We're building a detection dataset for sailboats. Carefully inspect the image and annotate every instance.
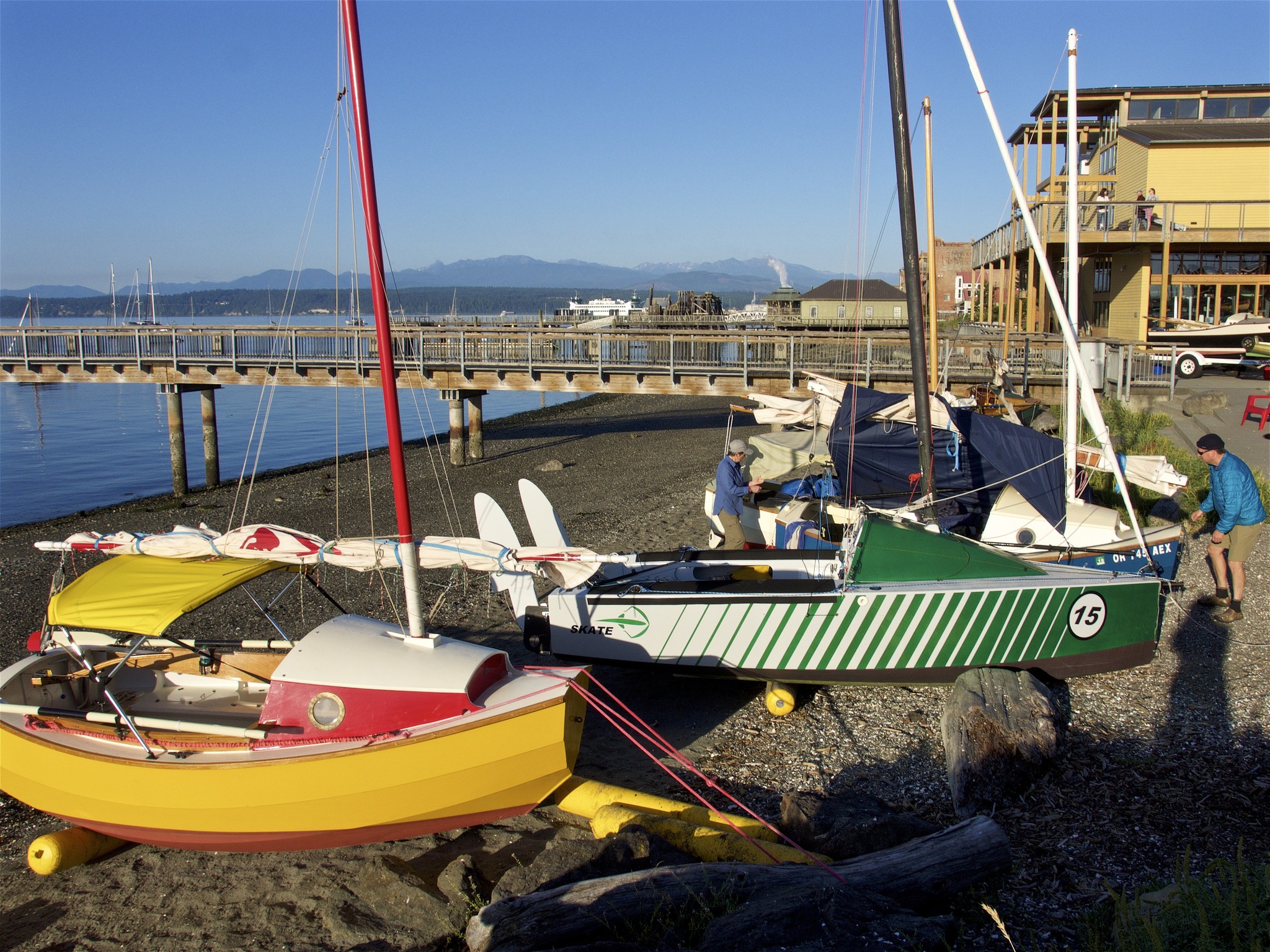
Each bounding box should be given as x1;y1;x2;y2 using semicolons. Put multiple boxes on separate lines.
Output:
0;0;595;854
469;0;1165;687
700;2;1187;594
6;257;183;354
343;269;367;326
188;296;202;334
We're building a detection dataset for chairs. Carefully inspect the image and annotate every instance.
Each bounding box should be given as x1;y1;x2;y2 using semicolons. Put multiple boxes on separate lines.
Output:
1240;395;1270;430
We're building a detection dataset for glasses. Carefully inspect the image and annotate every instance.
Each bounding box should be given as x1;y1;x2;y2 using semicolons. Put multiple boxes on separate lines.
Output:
1197;449;1211;455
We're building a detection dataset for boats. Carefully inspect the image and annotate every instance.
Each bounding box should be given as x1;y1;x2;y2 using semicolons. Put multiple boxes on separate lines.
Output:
382;275;645;329
1147;312;1270;348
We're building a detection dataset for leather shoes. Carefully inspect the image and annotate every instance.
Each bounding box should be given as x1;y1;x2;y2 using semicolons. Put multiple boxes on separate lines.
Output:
1197;594;1232;607
1213;608;1243;623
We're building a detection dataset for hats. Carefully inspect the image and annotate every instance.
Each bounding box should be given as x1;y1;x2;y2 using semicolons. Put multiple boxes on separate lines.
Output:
1196;433;1225;450
728;440;754;455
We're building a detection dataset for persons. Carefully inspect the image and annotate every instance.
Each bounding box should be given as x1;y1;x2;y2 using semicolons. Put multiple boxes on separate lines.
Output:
1133;189;1149;231
711;439;764;550
1189;432;1267;623
1095;188;1110;230
1142;187;1159;231
